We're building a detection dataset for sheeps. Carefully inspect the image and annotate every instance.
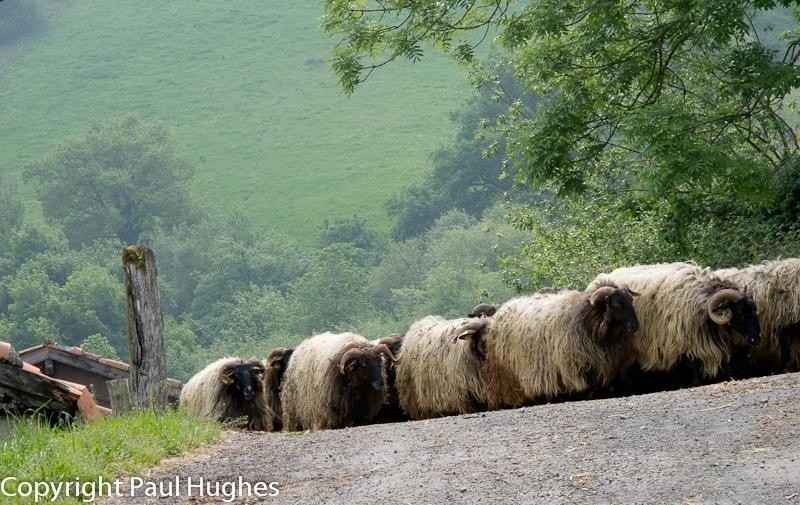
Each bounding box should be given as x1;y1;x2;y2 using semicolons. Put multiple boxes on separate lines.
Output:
278;330;405;438
483;260;641;414
609;258;800;397
176;346;293;436
394;302;497;421
